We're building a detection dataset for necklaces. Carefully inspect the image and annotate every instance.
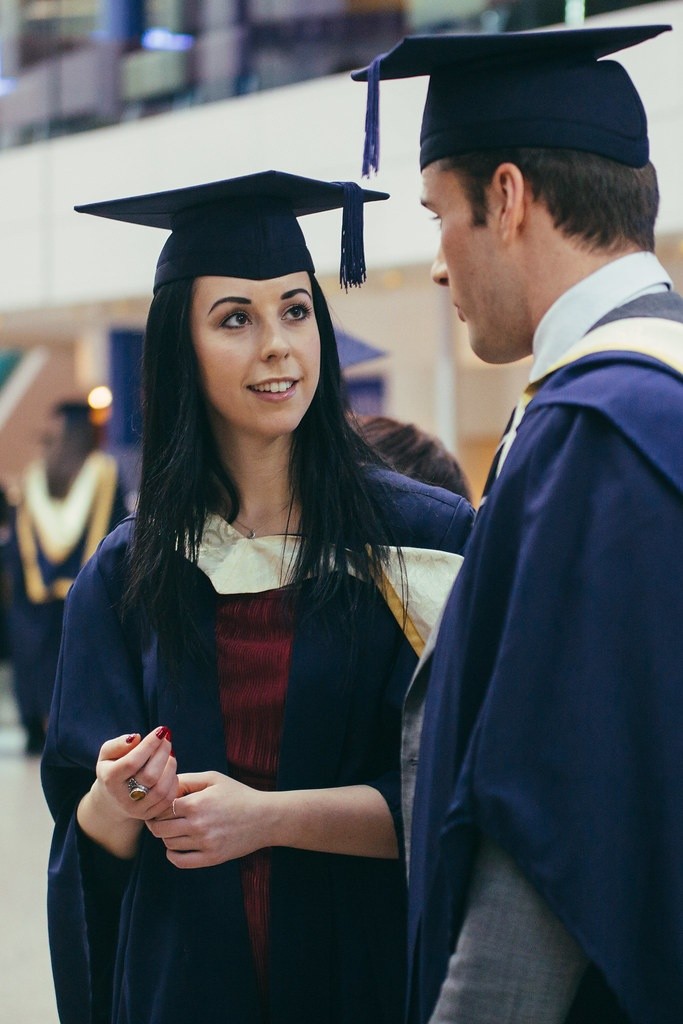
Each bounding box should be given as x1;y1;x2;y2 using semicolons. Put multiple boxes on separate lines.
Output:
236;500;290;538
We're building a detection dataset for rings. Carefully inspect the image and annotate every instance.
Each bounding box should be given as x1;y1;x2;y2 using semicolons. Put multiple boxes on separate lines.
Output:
172;797;177;816
127;778;150;802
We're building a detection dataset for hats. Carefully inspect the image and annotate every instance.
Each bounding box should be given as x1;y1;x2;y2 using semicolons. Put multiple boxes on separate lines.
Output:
75;169;390;294
351;24;672;179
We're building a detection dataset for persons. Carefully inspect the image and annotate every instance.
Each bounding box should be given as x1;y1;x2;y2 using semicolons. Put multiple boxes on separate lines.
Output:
38;172;476;1024
0;394;128;752
349;416;469;507
350;26;683;1024
